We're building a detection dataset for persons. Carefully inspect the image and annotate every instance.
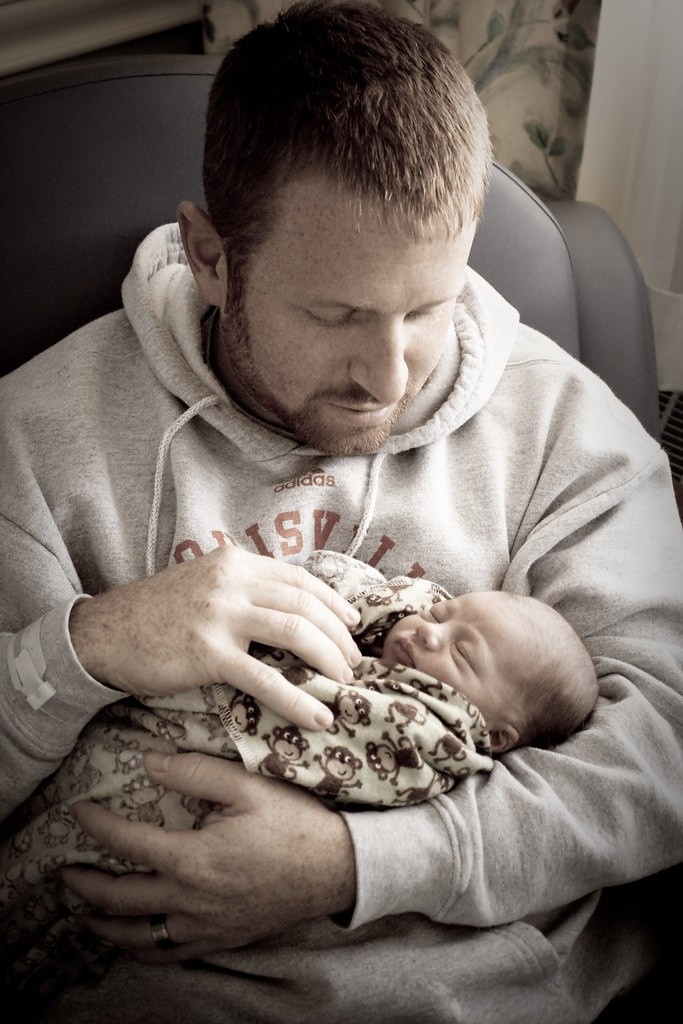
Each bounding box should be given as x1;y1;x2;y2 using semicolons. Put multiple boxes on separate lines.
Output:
1;0;683;1024
1;552;599;996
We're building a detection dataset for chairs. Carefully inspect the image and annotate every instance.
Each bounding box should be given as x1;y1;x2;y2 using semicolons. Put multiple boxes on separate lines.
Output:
0;53;658;446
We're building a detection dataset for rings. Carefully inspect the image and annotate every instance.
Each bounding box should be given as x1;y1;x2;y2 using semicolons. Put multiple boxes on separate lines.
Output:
150;915;183;949
